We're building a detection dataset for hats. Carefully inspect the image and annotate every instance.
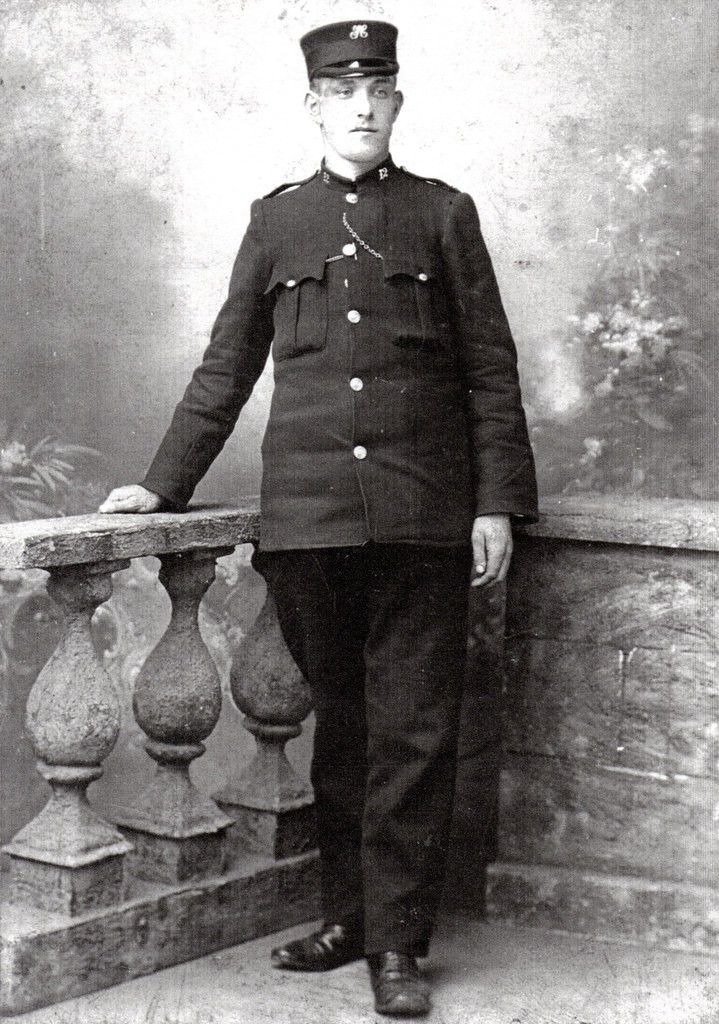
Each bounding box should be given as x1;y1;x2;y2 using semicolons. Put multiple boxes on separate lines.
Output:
299;20;400;82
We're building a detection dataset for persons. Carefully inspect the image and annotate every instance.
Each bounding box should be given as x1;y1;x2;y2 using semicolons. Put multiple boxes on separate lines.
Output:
99;21;542;1017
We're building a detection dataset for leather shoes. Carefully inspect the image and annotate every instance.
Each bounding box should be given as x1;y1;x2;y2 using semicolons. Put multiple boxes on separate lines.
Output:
365;952;430;1017
270;923;366;973
